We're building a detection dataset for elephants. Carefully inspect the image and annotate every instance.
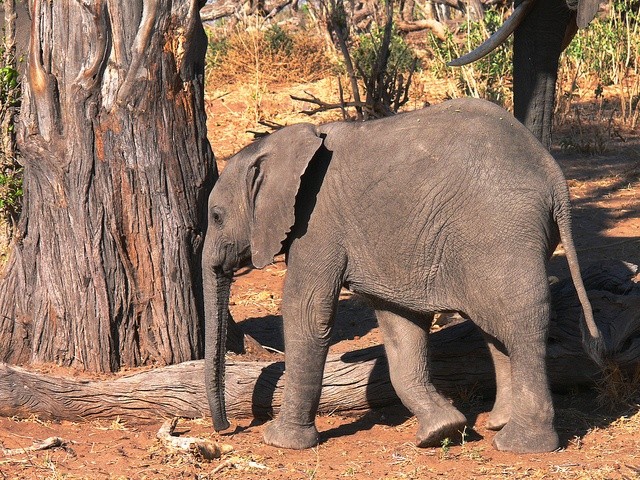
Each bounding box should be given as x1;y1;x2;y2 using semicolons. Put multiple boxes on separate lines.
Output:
200;96;609;454
